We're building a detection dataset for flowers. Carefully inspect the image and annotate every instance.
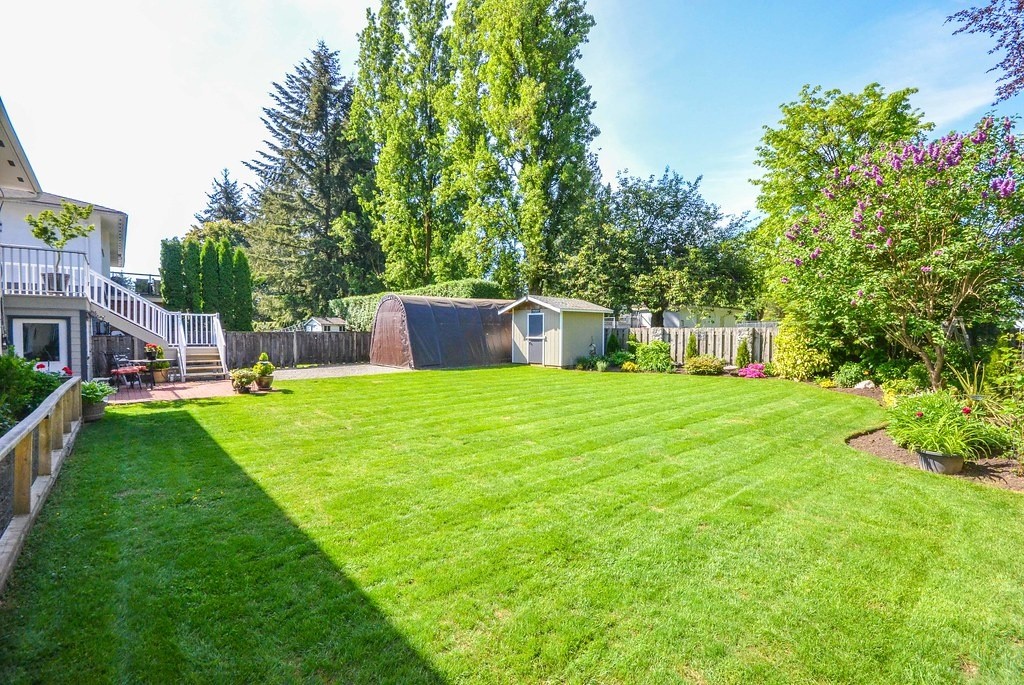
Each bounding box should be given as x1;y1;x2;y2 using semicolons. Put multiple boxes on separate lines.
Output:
144;343;161;354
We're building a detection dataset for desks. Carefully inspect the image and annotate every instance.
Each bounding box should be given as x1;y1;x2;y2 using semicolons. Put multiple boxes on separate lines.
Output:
128;359;178;390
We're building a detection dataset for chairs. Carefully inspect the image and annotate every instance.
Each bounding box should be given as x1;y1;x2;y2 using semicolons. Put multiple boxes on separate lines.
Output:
110;352;141;391
116;354;147;389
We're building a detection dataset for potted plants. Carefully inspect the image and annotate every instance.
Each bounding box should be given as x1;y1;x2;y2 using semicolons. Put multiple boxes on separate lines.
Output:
23;198;96;291
79;380;118;421
227;368;241;391
232;368;255;394
896;412;1002;476
146;361;171;383
253;353;275;391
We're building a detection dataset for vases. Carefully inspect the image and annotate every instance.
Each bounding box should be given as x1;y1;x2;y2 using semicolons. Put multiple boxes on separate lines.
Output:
147;351;158;360
